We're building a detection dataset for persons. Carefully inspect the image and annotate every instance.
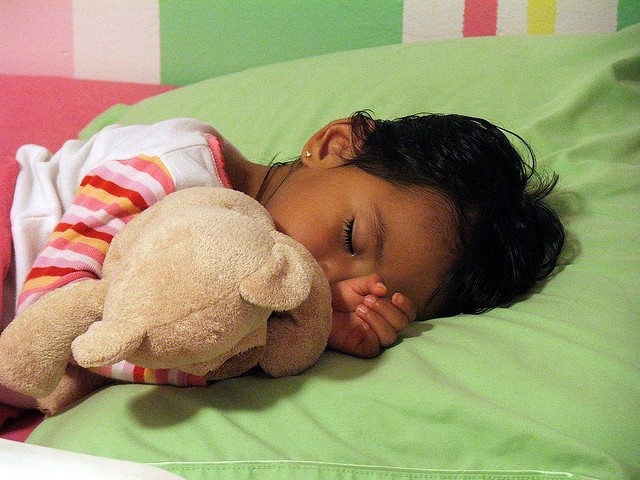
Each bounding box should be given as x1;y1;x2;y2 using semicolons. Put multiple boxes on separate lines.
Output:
1;109;566;421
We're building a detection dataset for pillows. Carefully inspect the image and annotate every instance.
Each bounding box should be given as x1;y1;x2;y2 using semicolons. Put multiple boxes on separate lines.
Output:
23;24;639;480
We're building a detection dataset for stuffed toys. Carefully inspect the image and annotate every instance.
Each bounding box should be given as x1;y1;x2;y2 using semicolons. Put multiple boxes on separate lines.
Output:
0;186;333;416
